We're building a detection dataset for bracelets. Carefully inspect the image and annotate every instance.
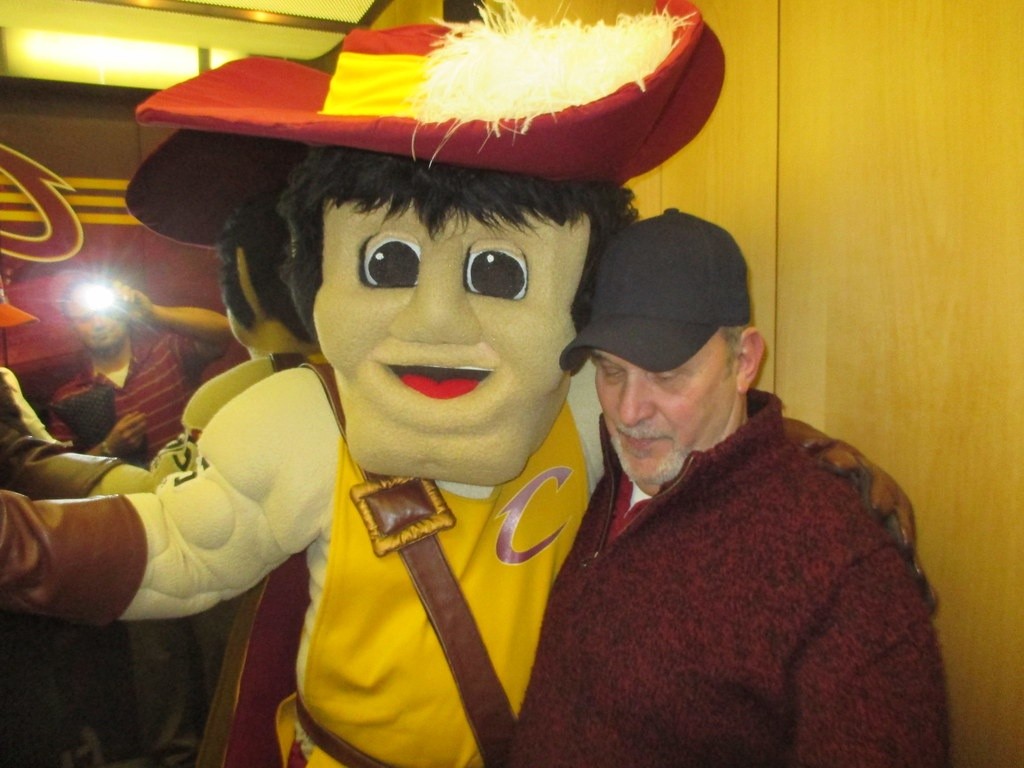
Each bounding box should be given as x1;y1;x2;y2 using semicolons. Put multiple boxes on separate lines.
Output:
100;440;114;457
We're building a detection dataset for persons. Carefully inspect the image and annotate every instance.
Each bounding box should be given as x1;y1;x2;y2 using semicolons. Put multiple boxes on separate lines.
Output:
0;119;321;765
45;260;230;764
505;206;958;768
0;1;925;768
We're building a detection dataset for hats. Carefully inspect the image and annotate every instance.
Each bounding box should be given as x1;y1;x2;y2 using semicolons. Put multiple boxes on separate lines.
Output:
560;208;751;377
